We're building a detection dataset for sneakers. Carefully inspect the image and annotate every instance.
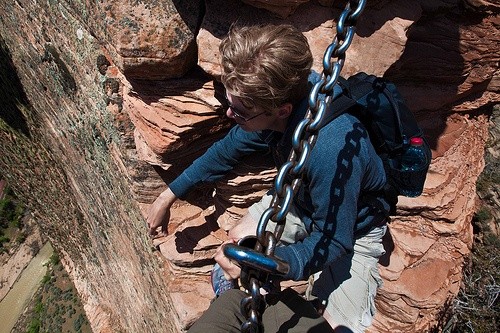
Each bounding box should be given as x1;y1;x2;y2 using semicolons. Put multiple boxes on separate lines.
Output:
210;262;238;297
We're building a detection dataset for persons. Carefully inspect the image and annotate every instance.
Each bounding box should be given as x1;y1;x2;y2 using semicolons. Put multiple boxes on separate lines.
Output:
186;263;335;333
145;21;391;333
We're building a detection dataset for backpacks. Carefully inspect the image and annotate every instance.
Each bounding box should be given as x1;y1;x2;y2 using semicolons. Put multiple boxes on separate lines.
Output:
318;73;432;197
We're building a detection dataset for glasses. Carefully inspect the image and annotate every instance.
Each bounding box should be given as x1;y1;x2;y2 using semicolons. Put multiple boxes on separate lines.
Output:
225;96;266;122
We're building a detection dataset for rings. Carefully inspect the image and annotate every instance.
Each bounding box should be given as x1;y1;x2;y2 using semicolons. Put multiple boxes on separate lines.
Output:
148;223;150;228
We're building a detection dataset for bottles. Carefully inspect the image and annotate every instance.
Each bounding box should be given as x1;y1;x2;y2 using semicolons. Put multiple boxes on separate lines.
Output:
400;138;427;198
